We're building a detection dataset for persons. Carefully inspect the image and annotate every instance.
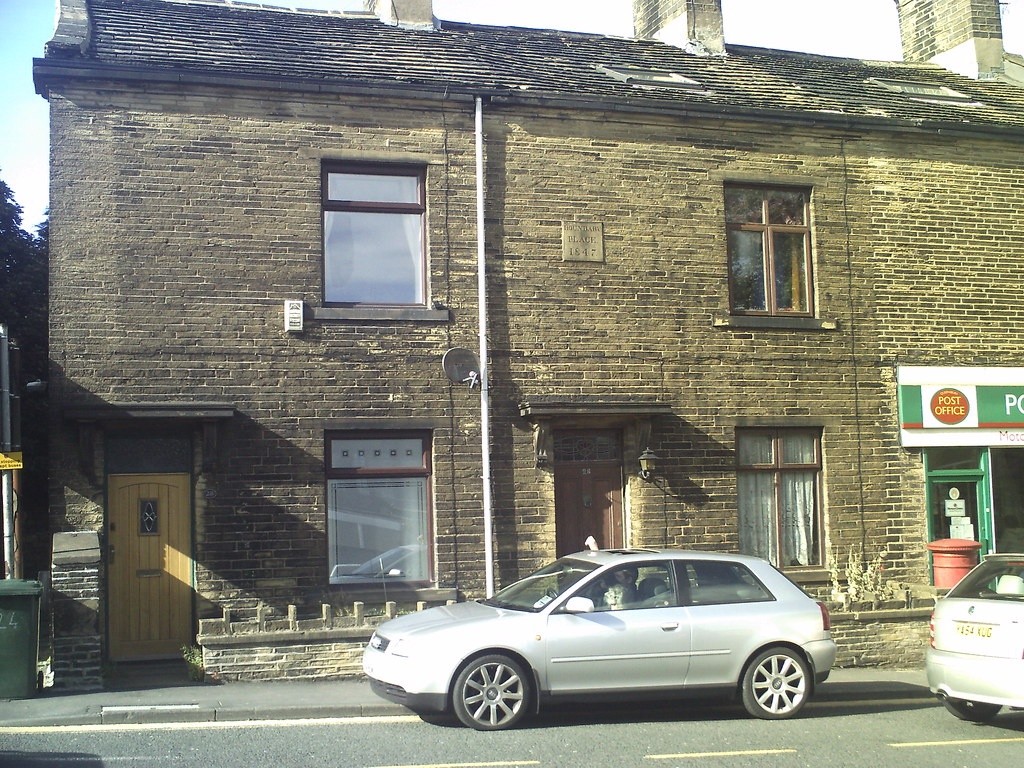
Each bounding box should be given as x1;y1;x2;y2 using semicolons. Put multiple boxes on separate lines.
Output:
996;515;1024;553
615;566;638;603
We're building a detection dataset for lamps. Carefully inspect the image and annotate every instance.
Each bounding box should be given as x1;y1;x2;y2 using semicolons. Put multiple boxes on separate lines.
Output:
638;446;663;480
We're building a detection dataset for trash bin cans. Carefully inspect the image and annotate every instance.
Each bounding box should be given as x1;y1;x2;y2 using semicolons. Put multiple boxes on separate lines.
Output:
0;579;44;698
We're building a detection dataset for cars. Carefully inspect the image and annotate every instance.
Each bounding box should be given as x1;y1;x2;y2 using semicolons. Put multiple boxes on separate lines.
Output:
362;547;836;731
924;552;1024;719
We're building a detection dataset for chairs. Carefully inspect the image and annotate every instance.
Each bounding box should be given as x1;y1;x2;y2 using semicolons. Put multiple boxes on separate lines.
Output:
634;578;668;607
603;569;639;605
